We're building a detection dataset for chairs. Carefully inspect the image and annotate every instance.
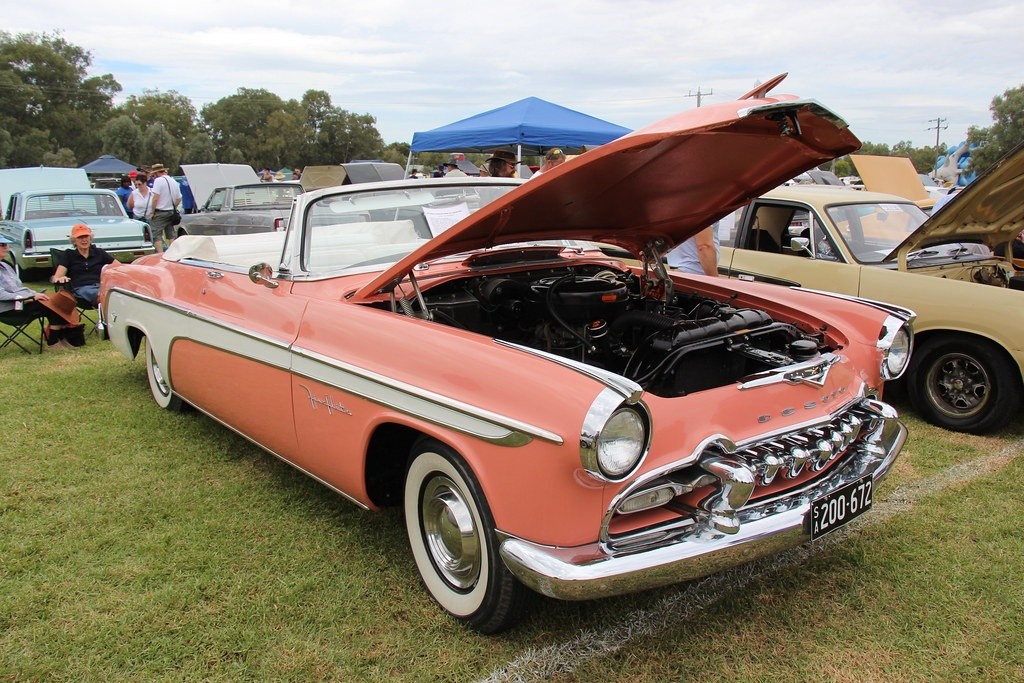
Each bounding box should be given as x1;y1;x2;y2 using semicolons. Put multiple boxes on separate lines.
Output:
748;229;781;253
0;288;47;355
50;244;96;336
274;197;296;206
801;227;829;256
234;199;252;208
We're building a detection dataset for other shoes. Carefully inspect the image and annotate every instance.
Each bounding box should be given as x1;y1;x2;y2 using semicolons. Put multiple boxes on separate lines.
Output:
50;338;75;349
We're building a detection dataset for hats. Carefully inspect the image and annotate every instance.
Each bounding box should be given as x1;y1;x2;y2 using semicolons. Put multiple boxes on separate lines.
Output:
484;151;524;165
0;234;15;243
443;157;458;168
72;224;91;239
150;164;170;174
36;291;79;324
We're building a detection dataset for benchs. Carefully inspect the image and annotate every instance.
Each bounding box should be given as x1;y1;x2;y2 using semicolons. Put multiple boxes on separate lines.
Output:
163;220;432;274
26;209;96;220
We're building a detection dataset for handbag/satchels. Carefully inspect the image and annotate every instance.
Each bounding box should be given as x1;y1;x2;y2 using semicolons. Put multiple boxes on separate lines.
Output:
138;217;146;222
171;212;181;225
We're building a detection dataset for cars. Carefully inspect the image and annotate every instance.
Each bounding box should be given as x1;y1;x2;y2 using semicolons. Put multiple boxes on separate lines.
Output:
0;161;480;283
716;141;1024;435
97;73;917;636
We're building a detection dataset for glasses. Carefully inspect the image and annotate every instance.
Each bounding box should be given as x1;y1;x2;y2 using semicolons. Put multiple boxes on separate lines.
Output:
548;149;563;159
0;243;6;247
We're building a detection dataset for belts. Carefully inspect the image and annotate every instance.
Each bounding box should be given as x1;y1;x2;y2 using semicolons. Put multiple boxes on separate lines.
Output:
155;208;174;212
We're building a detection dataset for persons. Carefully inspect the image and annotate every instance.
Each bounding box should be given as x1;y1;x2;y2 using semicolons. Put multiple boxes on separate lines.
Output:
409;169;418;179
0;235;74;351
443;159;468;177
129;165;154;189
293;173;298;180
51;224;123;307
438;164;445;177
434;172;440;177
127;174;153;223
116;176;134;219
149;164;182;253
668;219;720;277
485;150;524;178
530;148;566;179
261;168;272;182
293;168;301;179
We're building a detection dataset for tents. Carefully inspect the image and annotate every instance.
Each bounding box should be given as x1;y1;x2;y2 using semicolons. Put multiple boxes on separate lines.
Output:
257;169;276;175
280;167;294;174
391;96;636;221
80;155;139;173
